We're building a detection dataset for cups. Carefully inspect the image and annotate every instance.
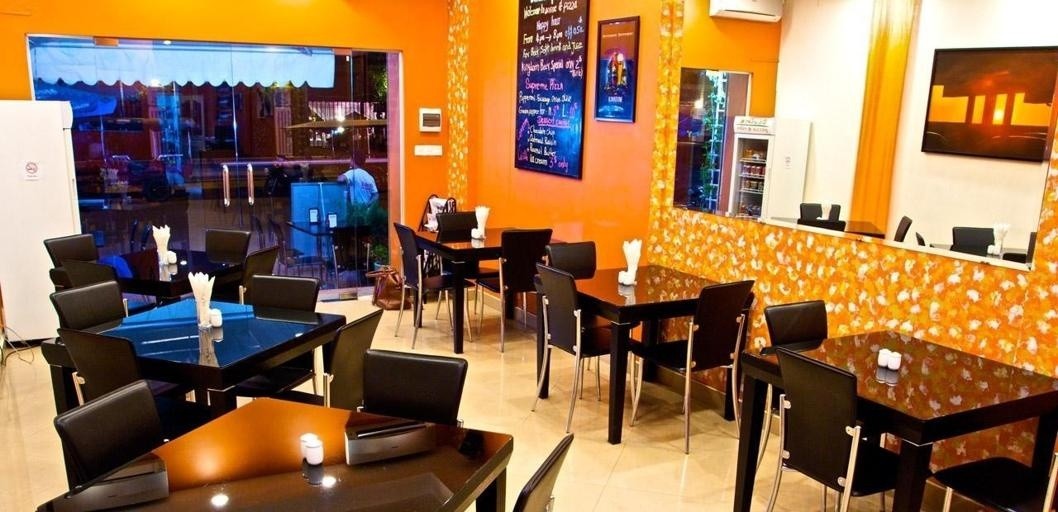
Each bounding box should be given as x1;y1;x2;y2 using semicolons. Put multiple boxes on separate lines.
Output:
470;222;488;239
298;432;330;469
195;298;221;329
158;250;176;265
618;259;640;287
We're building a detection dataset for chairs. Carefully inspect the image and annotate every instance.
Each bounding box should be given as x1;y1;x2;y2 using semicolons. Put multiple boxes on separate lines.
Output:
532;241;756;455
734;298;1057;511
31;199;574;512
798;202;1037;266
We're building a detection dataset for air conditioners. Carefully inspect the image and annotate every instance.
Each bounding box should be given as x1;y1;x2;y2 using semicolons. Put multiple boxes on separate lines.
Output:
708;0;784;25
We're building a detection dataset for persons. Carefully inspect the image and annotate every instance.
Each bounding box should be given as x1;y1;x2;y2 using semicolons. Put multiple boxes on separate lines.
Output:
338;144;380;208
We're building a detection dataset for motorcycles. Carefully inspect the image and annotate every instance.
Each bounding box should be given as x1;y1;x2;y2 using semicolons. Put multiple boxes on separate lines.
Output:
262;151;314;196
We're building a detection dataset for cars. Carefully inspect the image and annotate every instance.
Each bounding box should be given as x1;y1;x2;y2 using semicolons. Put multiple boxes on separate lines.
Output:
158;153;191;166
109;153;133;165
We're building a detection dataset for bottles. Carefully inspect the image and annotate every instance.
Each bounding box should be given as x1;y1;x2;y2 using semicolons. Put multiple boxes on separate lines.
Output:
877;349;901;370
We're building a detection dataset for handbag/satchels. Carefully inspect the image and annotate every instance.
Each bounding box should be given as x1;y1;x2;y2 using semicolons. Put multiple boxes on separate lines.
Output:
371;263;412;311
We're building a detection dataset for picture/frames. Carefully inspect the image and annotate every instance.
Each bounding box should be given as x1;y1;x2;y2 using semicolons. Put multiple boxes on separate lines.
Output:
919;43;1057;164
593;13;641;124
